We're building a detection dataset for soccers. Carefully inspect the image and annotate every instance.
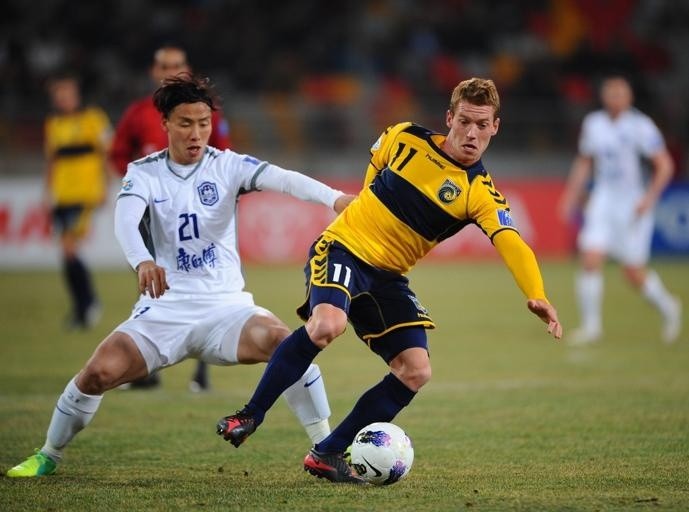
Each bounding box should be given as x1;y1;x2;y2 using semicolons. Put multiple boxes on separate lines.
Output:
351;422;414;484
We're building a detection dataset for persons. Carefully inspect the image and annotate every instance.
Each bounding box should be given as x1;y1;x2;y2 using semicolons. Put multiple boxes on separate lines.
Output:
557;78;682;342
216;77;562;483
6;72;360;478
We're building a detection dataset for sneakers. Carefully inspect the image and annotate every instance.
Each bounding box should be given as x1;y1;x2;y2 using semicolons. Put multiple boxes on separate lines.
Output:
304;448;365;482
216;410;257;448
6;448;59;477
